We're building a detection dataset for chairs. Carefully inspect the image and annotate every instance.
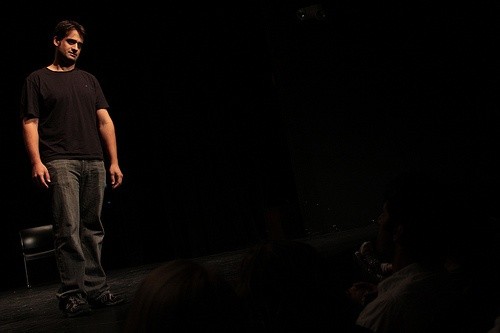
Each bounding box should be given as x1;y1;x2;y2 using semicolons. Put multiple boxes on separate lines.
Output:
20;224;62;288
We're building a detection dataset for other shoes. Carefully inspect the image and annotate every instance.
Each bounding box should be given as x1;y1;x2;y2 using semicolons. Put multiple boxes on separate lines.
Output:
58;293;94;318
89;289;124;309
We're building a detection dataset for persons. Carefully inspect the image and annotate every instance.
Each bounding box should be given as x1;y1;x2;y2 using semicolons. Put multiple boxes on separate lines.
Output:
125;187;462;333
24;20;123;316
